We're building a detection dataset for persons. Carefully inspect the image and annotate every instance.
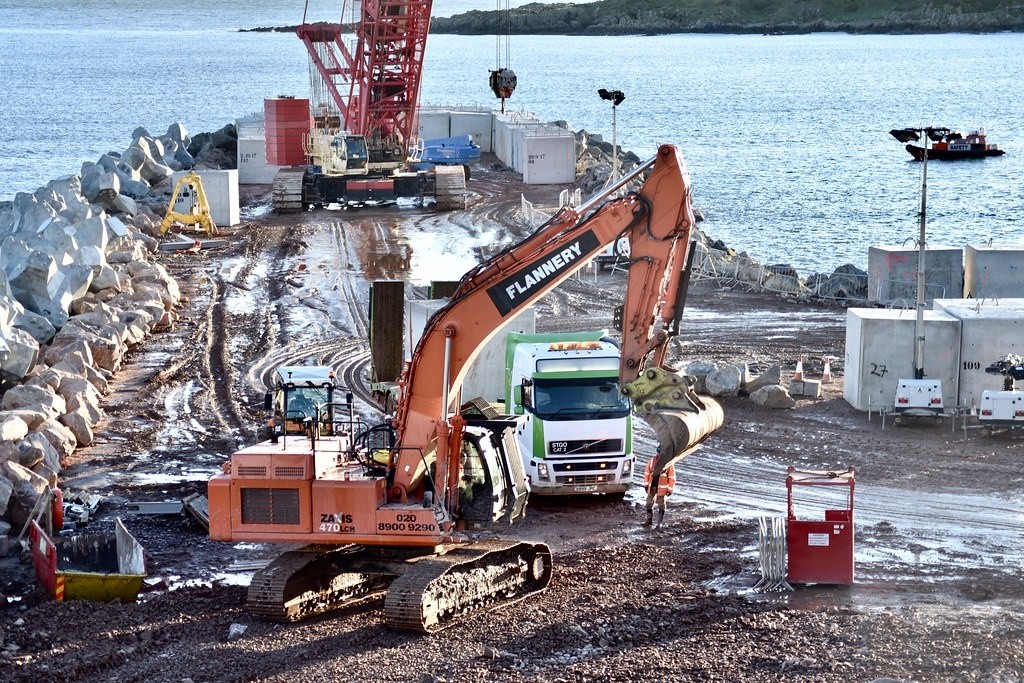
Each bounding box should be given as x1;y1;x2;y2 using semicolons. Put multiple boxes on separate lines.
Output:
642;446;675;532
447;452;467;507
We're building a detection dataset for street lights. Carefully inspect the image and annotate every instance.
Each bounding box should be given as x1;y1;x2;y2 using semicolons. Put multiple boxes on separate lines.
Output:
596;88;626;200
889;124;951;380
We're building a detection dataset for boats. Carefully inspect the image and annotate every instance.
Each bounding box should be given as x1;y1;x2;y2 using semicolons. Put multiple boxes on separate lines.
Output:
904;126;1005;162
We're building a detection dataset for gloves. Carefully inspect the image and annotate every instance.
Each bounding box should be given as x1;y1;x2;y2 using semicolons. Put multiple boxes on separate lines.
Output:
645;487;648;493
667;492;671;496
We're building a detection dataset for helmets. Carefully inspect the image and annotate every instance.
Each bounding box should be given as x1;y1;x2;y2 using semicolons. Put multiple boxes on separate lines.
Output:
656;446;660;452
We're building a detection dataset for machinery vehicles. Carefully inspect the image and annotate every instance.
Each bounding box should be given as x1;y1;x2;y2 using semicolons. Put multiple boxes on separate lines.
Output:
268;0;518;213
207;145;725;636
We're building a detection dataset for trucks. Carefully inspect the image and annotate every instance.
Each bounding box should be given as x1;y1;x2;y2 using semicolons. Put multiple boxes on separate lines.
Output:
403;298;633;505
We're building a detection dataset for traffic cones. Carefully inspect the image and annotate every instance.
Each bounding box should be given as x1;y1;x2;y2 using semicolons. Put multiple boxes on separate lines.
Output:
821;356;835;384
791;352;807;383
586;262;594;275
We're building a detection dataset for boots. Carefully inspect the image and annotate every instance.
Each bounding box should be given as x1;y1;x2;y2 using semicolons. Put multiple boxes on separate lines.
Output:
641;509;653;525
657;510;665;533
